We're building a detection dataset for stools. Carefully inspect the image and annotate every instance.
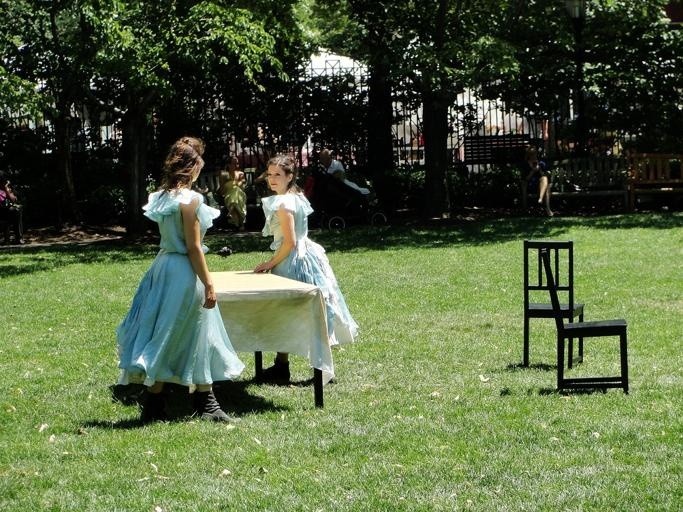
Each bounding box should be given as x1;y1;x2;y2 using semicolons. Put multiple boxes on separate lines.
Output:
0;220;17;245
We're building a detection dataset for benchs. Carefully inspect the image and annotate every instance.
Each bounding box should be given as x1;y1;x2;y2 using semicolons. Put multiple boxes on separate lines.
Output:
520;156;629;216
626;151;683;212
192;168;275;234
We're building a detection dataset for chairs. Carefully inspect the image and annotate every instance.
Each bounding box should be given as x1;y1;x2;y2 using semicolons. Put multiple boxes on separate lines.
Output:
523;241;583;369
541;247;628;395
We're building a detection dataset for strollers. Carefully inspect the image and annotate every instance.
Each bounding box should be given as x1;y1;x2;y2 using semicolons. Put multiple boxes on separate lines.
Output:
311;164;388;234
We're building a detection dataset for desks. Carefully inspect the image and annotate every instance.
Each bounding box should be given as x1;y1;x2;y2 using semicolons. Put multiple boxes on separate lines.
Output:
197;271;335;410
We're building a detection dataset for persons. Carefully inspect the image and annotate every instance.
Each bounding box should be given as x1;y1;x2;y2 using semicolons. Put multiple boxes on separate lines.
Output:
0;170;25;245
185;137;375;234
114;137;245;420
250;154;336;387
520;145;555;219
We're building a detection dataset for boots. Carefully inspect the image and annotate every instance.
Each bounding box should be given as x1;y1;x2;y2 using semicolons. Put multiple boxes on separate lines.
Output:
295;377;333;385
262;358;290;382
199;391;242;423
140;391;176;422
